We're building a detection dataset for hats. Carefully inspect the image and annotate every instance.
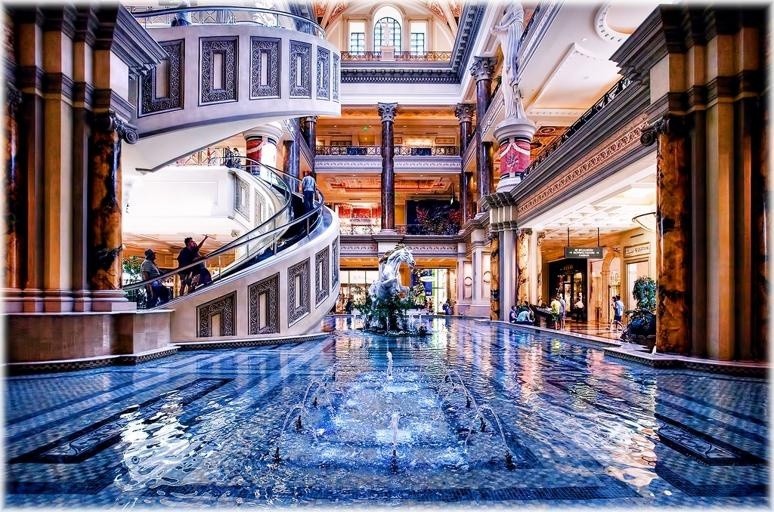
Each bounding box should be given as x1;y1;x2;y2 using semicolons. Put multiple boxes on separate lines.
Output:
144;249;155;259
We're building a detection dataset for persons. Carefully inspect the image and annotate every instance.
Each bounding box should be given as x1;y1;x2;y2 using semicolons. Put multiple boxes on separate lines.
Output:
225;146;240;168
365;292;372;306
175;0;187;26
489;0;527;121
510;293;566;330
176;235;212;296
613;295;624;328
86;243;119;290
575;298;584;322
301;171;316;212
140;249;169;309
346;298;353;314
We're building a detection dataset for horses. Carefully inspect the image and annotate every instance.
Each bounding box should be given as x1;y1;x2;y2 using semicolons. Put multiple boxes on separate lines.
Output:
375;243;416;304
